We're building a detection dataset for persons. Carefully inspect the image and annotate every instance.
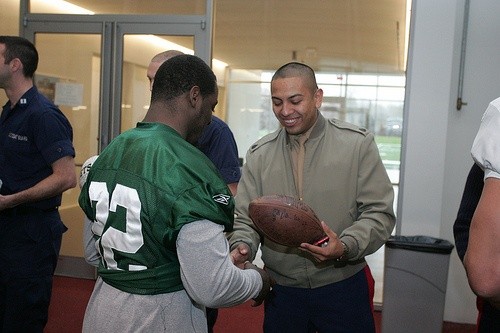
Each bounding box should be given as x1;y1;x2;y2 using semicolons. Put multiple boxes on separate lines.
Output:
146;49;241;332
0;35;78;333
452;97;500;332
226;61;396;333
75;54;269;333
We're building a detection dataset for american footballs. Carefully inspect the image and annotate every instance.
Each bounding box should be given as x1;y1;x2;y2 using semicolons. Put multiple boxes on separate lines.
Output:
249;193;324;247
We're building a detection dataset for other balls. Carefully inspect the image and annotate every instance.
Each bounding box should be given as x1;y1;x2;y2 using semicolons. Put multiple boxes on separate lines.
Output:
78;155;100;190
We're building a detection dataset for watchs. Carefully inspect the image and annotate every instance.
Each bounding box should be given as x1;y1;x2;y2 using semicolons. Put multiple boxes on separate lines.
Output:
336;241;350;269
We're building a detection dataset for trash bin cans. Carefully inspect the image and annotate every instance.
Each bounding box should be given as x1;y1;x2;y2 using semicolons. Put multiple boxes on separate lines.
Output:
380;235;455;333
